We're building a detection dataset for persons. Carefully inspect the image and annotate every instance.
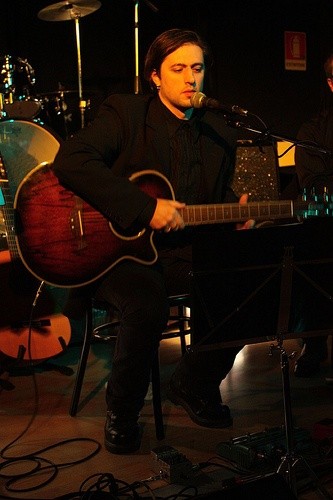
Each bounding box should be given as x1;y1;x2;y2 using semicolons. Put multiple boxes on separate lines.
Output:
53;29;275;457
289;57;332;376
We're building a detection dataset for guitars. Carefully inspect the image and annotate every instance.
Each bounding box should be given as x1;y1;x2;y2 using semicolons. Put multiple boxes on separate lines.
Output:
0;153;71;361
13;158;333;289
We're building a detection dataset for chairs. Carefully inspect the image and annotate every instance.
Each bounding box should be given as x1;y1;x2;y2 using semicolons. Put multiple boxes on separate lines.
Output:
69;282;194;440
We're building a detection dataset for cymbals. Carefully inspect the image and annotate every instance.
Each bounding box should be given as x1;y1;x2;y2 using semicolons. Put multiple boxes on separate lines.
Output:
38;0;101;22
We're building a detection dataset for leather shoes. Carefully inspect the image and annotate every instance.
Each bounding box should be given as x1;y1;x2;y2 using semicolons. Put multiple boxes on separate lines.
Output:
104;410;141;455
166;377;234;429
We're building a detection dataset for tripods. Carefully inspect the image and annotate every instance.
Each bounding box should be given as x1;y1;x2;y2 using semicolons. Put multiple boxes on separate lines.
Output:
180;212;333;500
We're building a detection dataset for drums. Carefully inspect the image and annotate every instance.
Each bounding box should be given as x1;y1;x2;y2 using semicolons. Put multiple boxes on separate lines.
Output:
1;119;66;238
0;55;44;120
35;90;95;141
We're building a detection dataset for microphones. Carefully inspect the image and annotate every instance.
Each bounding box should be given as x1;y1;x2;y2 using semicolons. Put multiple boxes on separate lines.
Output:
189;91;249;117
